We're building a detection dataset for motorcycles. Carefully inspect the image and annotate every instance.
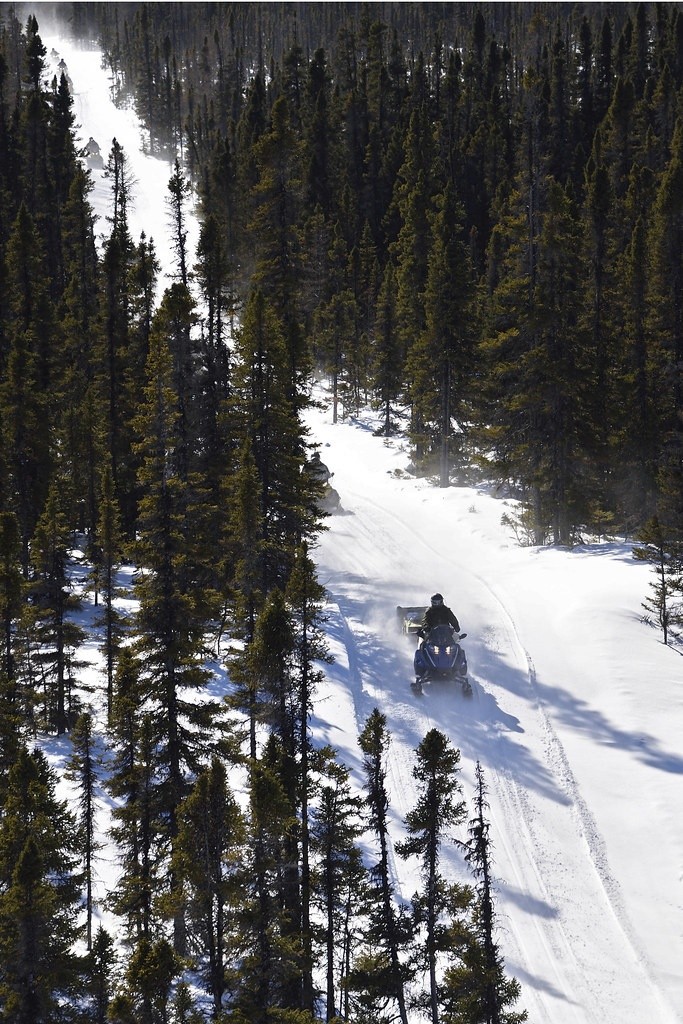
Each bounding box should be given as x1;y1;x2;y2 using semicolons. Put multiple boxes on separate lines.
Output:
309;470;346;518
409;615;473;699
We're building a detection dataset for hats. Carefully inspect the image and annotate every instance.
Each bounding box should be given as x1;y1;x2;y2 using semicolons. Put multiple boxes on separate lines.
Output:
431;594;443;600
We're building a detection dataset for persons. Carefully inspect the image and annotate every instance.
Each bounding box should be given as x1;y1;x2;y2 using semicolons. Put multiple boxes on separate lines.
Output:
423;593;461;639
302;452;330;483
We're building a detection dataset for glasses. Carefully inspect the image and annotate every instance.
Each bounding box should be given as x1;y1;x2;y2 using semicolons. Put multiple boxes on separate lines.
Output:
431;601;437;604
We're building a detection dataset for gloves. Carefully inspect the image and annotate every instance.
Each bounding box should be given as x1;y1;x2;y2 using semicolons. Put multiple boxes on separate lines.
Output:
453;626;460;632
424;625;431;632
